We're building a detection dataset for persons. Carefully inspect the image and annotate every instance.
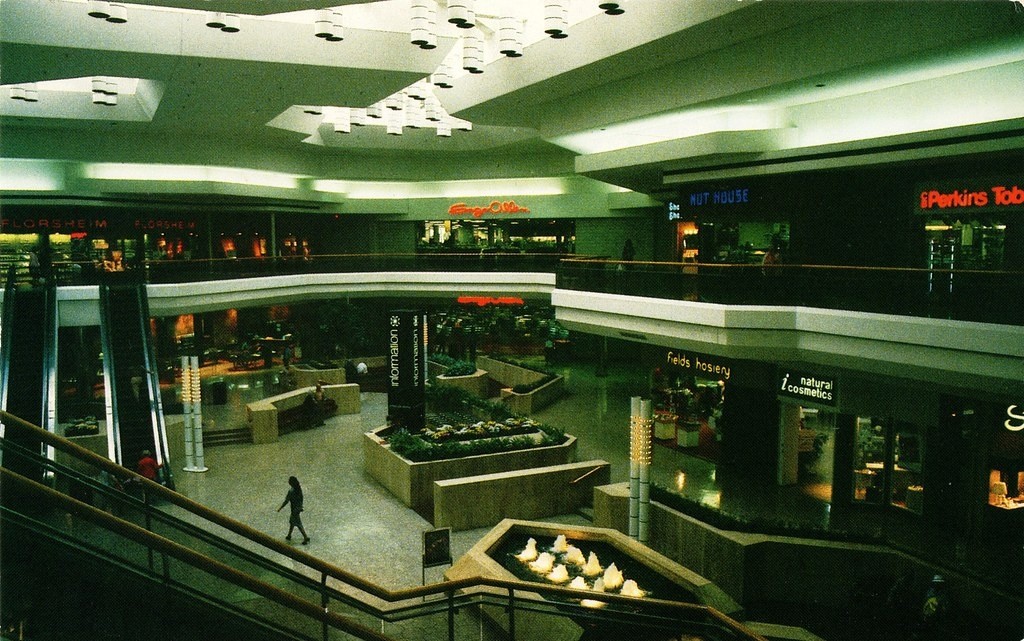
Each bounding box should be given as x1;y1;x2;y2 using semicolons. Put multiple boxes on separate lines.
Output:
278;476;310;545
136;450;162;483
357;362;368;374
29;246;42;286
303;384;327;430
127;361;154;402
284;344;293;367
434;325;480;365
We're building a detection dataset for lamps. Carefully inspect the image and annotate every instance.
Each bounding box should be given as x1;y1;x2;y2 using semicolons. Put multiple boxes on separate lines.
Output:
989;482;1007;506
7;0;624;138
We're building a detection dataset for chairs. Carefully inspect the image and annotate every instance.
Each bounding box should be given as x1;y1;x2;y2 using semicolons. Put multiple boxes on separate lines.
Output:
344;362;387;394
175;329;296;370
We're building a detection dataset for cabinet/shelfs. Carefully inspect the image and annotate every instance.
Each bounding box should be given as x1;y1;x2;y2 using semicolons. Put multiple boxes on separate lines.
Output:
0;247;135;284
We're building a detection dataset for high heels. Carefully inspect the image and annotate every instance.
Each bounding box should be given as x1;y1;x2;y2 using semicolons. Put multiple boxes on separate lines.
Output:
286;536;291;541
302;537;310;545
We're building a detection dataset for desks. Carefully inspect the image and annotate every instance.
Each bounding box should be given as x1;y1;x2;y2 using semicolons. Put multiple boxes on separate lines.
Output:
988;499;1024;511
652;383;922;513
